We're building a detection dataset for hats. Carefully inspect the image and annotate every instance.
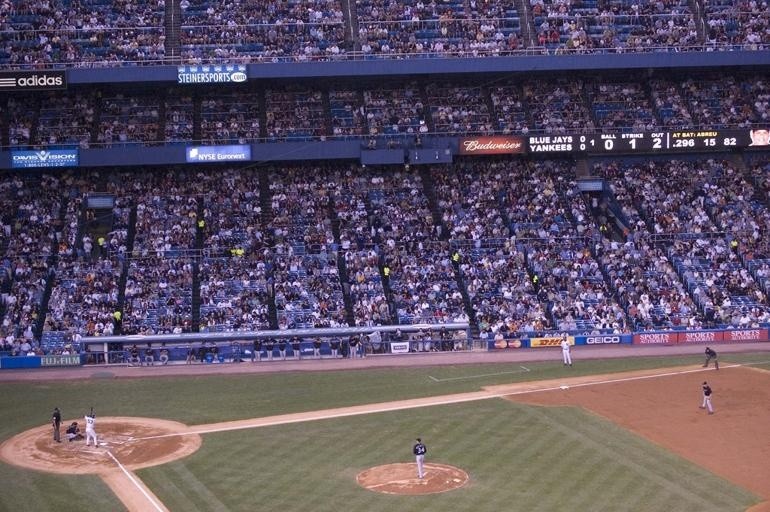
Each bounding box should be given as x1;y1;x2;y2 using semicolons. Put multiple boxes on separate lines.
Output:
705;347;709;351
416;438;421;443
54;407;59;410
72;422;77;426
702;381;707;385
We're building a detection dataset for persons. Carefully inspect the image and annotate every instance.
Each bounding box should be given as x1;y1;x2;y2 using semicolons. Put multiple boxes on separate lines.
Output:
699;381;714;414
66;422;84;442
703;346;721;371
84;411;100;448
1;1;769;360
559;336;573;366
413;438;427;479
52;408;64;443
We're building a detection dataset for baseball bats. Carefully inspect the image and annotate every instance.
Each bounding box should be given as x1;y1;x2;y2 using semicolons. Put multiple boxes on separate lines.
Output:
90;406;93;417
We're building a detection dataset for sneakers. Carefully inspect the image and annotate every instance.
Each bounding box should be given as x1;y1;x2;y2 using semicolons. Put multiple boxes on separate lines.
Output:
87;443;98;448
418;474;424;479
54;438;61;442
699;406;714;415
702;365;719;370
564;363;572;367
